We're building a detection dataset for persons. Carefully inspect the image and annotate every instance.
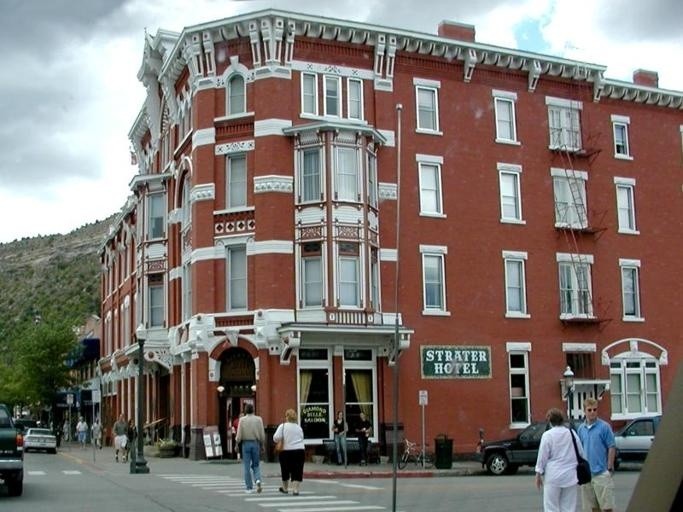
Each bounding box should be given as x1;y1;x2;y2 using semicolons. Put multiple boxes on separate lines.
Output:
13;413;137;463
232;411;246;464
329;409;350;466
234;403;265;494
273;409;306;495
534;407;584;512
577;397;617;512
354;411;373;467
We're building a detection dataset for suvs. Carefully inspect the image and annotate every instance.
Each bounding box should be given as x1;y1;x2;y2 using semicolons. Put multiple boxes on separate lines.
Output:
15;418;38;434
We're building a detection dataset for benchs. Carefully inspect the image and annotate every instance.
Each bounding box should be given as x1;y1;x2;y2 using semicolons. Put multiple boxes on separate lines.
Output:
320;439;382;467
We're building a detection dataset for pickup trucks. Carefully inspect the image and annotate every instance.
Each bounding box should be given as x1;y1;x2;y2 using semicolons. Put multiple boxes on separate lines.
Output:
0;404;24;497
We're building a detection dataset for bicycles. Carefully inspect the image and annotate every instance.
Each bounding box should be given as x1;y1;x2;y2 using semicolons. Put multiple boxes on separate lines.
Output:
399;438;436;472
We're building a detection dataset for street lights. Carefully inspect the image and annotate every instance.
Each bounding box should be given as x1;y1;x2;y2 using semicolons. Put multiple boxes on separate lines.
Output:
132;324;149;473
563;366;576;431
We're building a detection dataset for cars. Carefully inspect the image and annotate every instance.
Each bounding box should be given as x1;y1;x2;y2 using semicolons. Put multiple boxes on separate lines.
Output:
613;415;659;470
481;418;620;475
24;428;57;453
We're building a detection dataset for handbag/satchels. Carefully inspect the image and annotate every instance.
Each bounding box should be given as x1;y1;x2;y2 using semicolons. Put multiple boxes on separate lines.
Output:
274;440;282;454
577;457;591;485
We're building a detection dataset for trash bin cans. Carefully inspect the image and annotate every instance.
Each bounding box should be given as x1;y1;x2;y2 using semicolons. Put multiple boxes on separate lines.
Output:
435;433;453;469
264;424;279;462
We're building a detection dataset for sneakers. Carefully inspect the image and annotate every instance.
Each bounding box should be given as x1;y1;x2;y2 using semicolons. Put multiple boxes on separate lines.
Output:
256;480;261;493
279;487;288;492
